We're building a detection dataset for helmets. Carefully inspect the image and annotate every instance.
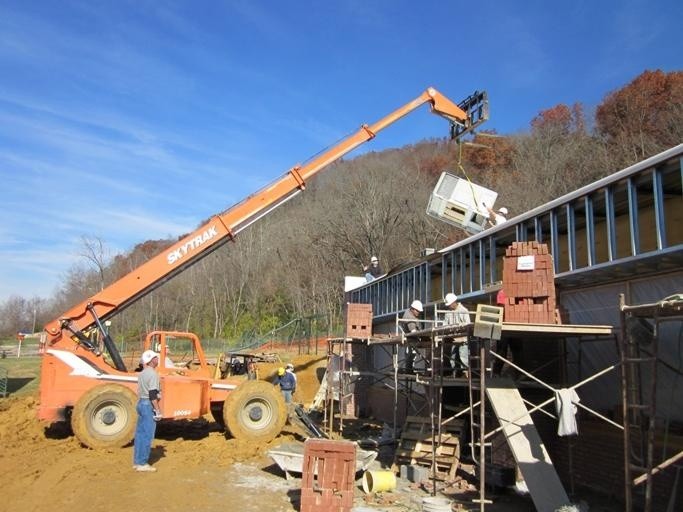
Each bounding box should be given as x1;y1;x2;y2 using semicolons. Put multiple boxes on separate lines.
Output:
141;350;160;364
157;343;168;352
496;207;508;215
276;367;285;376
443;293;458;306
233;359;239;364
410;300;424;313
370;256;378;263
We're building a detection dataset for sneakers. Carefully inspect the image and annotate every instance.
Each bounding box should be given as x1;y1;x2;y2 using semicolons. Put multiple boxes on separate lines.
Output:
132;463;157;473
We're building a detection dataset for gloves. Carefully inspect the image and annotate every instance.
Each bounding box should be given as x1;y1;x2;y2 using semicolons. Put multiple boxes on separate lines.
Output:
152;409;162;421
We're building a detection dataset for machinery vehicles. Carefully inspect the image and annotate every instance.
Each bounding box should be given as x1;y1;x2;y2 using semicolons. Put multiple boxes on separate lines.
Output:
38;86;489;450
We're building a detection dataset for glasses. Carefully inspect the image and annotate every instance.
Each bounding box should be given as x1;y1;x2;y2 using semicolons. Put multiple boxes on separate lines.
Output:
372;260;379;262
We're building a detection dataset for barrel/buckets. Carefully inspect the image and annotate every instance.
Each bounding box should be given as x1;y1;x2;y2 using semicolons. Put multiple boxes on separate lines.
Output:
362;470;395;494
422;498;452;512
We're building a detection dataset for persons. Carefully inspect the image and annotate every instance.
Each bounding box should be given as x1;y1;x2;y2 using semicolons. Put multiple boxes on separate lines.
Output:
364;256;382;282
442;292;471;379
486;207;508;227
134;360;143;372
132;350;162;472
285;364;297;393
231;359;245;377
401;300;425;374
156;344;175;368
490;288;522;380
272;367;296;404
